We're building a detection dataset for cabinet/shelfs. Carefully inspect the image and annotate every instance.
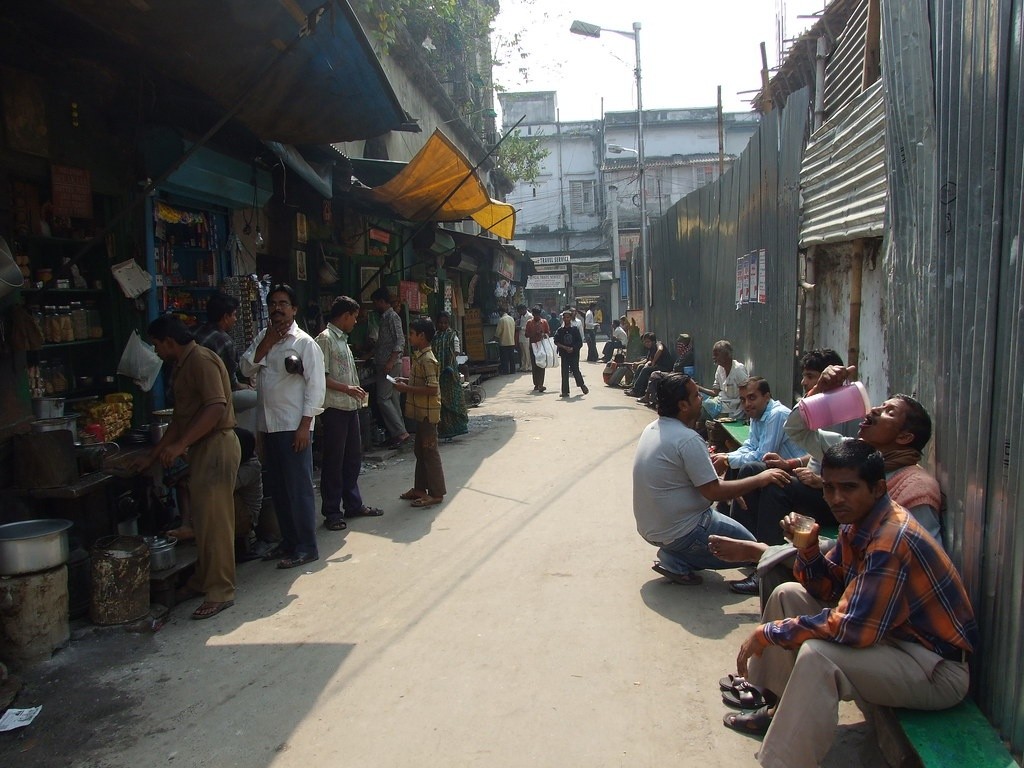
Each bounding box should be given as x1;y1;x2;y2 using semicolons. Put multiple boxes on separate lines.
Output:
156;199;231;328
19;182;144;430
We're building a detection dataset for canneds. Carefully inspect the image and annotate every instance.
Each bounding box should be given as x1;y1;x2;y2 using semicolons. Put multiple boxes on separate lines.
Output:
191;298;207;310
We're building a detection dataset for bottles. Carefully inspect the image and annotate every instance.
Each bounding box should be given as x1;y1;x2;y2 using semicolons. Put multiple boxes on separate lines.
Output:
30;300;102;344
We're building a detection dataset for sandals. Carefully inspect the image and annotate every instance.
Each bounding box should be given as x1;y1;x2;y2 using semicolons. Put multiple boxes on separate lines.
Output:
344;504;384;518
723;704;773;736
262;545;319;568
325;518;347;531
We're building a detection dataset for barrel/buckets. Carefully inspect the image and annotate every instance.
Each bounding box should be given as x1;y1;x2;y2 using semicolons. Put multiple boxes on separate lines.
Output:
0;249;24;299
31;414;81;443
91;534;151;627
32;398;65;418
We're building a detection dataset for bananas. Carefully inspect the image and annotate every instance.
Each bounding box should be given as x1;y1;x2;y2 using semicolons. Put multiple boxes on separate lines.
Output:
419;282;433;295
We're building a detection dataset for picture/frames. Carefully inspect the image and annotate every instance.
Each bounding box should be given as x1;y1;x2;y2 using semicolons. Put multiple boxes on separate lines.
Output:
296;212;307;244
296;250;307;281
360;266;380;304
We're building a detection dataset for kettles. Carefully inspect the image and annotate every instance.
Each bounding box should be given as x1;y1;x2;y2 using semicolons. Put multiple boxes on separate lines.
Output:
145;534;177;571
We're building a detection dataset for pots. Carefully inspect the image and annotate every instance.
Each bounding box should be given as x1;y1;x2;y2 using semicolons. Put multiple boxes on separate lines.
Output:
117;513;140;537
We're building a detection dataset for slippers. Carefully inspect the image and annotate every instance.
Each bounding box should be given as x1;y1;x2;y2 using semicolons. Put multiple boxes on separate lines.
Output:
387;432;416;450
191;600;235;619
719;673;775;709
652;561;703;584
176;586;208;603
400;488;443;506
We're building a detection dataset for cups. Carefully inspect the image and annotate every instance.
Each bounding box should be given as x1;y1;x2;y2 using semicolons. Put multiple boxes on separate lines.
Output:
360;392;369;408
793;514;815;548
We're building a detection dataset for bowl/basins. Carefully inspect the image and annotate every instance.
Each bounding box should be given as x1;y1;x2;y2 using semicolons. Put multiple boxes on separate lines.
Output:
0;519;73;575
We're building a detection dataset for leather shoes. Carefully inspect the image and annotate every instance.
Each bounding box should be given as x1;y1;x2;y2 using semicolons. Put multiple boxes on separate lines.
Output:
727;568;762;596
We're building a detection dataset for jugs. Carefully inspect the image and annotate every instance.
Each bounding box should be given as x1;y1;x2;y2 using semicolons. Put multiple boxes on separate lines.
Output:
798;379;872;430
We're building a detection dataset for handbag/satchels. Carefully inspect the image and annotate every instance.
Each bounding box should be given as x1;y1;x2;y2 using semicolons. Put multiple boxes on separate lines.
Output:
116;328;164;392
531;333;560;368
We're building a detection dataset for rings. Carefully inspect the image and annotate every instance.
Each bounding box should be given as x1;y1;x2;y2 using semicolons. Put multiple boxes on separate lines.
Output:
276;327;282;337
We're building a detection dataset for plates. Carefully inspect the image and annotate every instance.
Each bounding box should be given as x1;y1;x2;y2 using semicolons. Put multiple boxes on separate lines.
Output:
124;427;151;443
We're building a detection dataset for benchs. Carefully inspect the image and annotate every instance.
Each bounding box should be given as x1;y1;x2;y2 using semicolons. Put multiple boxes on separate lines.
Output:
819;527;1020;768
699;392;750;445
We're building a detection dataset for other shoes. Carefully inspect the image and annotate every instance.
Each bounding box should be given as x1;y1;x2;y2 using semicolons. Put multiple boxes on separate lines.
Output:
637;397;649;403
624;388;636;397
581;385;590;395
534;386;547;393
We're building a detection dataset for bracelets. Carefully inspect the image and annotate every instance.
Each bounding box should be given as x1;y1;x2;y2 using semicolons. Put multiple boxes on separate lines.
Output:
796;458;804;468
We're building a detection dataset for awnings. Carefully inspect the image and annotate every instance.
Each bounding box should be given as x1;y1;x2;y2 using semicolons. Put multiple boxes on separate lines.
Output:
351;126;516;241
0;0;410;144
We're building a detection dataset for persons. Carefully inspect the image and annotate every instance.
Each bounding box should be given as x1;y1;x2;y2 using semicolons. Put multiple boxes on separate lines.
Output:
495;303;600;397
695;340;749;443
0;287;41;524
394;319;447;508
167;427;263;556
710;348;866;595
431;311;470;440
632;372;791;585
718;392;979;768
200;293;256;391
371;286;410;450
239;283;327;569
313;295;384;531
127;315;242;620
600;315;694;407
